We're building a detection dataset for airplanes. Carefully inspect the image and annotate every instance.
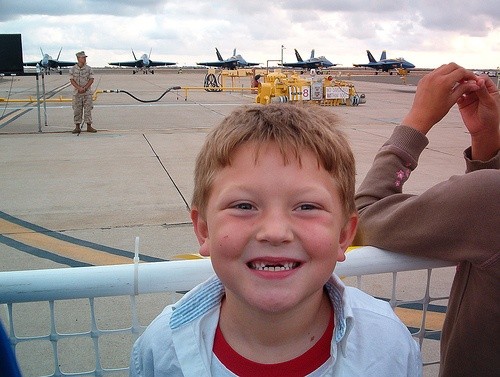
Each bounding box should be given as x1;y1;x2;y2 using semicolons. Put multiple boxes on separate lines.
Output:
23;46;87;75
108;47;176;75
277;48;337;71
353;49;416;75
196;47;259;70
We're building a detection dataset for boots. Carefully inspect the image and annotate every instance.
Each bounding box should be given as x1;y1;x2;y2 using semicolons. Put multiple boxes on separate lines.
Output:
86;124;97;133
72;124;81;133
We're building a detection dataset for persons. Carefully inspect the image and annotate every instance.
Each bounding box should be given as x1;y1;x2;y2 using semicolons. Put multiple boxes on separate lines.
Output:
70;51;97;134
127;100;421;377
354;63;500;376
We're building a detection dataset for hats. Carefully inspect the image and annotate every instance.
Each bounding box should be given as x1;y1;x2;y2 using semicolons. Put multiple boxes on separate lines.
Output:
76;51;88;58
